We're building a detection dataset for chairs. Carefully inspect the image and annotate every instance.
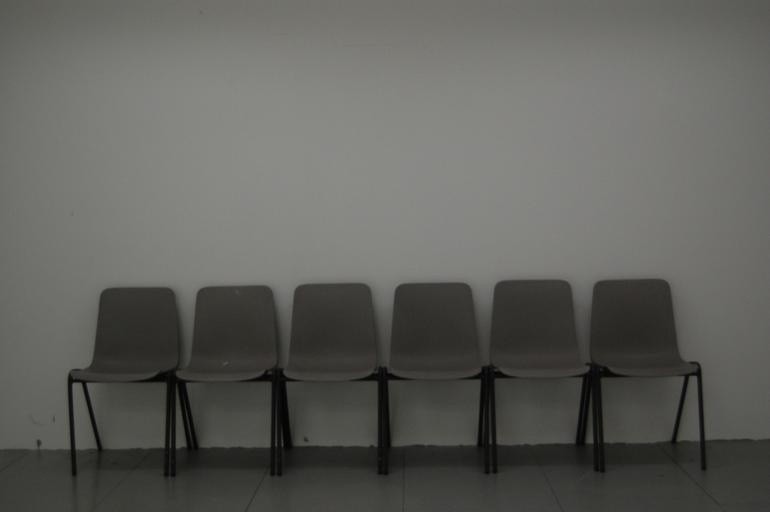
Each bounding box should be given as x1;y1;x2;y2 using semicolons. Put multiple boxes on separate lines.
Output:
168;279;286;481
484;279;600;479
579;274;710;474
64;281;200;483
382;278;495;481
273;277;393;483
